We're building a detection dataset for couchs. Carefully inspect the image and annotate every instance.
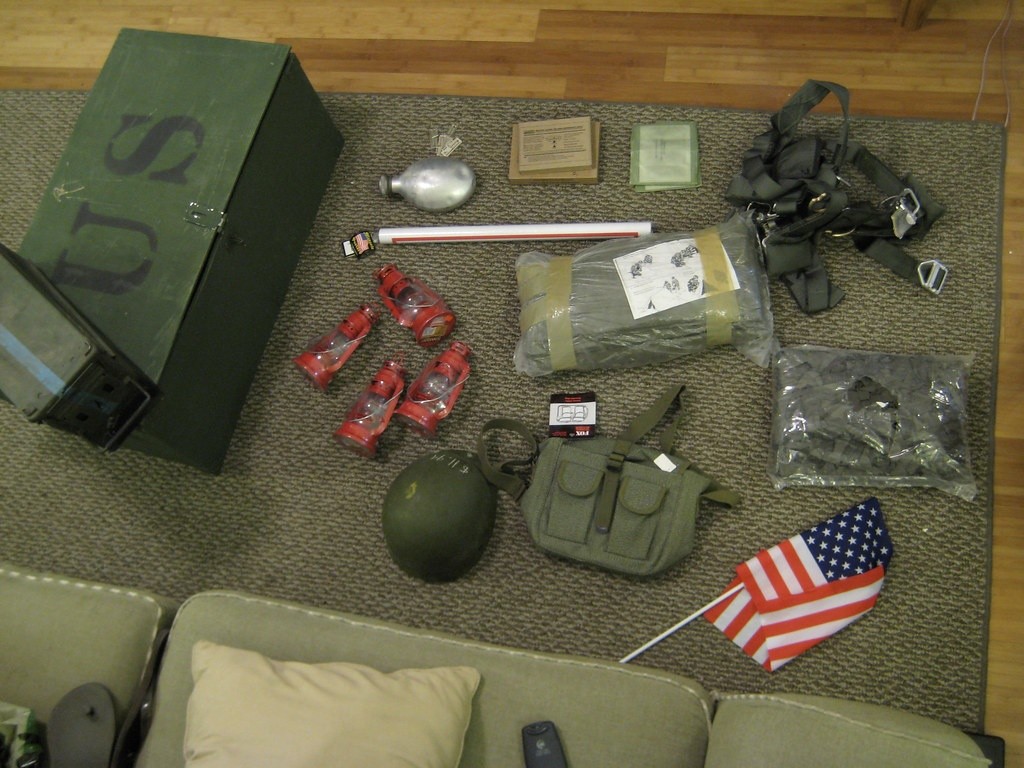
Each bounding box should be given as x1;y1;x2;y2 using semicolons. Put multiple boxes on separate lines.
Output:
0;558;991;768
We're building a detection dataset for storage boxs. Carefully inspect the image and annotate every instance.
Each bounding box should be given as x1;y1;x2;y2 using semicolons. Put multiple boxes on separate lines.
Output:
1;28;343;476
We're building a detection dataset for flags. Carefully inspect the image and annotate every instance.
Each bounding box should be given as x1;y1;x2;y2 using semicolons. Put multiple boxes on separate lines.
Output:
702;495;895;673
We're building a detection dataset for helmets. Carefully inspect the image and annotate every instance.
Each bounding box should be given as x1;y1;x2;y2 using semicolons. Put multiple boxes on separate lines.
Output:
382;452;496;584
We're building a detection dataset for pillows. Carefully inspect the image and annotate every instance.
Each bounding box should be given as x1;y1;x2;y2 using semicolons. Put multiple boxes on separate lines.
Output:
184;639;481;768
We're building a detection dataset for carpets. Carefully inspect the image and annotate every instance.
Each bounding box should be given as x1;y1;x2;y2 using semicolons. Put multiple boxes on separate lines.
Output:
1;88;1007;732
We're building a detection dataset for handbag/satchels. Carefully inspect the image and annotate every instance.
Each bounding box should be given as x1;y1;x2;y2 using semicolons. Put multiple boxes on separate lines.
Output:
520;434;711;577
